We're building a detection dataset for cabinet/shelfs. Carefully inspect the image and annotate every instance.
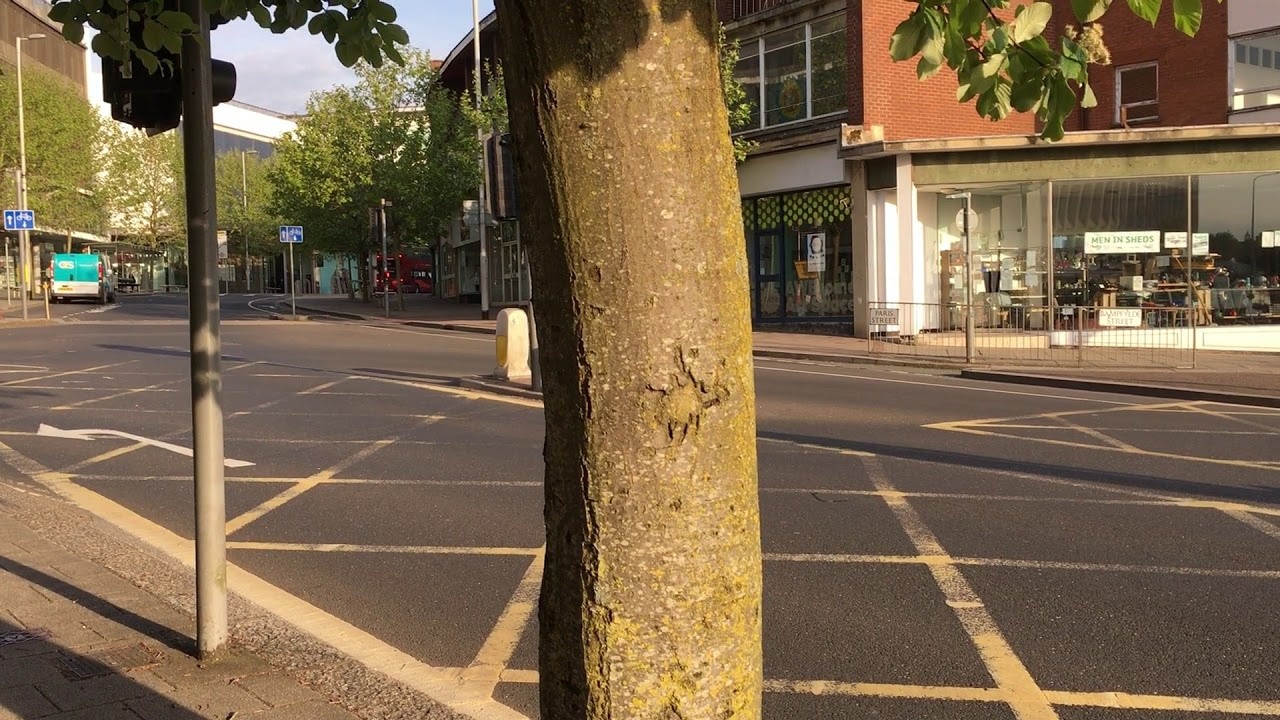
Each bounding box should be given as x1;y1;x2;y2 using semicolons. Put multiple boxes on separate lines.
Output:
940;246;1211;327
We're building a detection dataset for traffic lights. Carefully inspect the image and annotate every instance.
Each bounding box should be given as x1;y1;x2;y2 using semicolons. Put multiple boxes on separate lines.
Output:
101;0;237;135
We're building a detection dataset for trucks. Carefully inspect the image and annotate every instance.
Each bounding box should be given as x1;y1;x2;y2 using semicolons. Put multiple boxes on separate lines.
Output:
374;253;433;293
46;247;119;304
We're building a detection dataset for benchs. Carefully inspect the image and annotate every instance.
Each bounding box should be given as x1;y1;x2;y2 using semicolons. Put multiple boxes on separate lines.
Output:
161;284;185;292
119;283;141;293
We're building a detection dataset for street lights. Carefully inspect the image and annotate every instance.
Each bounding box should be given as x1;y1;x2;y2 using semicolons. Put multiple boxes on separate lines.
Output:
945;191;976;364
241;150;258;293
16;33;47;297
